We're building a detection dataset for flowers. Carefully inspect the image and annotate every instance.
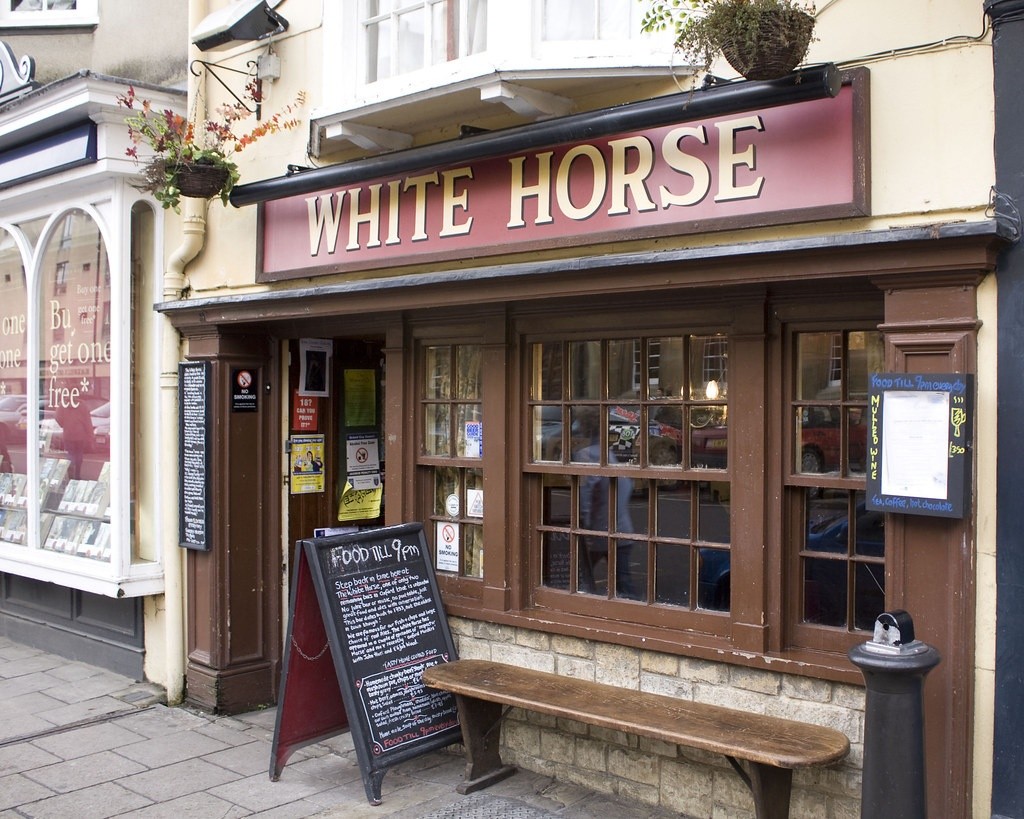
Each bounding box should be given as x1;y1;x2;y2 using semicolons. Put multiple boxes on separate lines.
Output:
638;0;825;110
117;77;308;216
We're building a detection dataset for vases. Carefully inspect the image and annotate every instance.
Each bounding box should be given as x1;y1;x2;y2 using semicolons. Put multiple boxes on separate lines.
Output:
178;166;226;200
723;13;813;81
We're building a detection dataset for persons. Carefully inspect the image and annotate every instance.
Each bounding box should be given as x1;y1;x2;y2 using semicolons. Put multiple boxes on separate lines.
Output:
848;408;863;426
54;385;95;480
0;422;13;473
573;406;637;600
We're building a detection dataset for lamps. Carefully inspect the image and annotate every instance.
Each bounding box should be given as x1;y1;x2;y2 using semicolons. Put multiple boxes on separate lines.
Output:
188;0;290;52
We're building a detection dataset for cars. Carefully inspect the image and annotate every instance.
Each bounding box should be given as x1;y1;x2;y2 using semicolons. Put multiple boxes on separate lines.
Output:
543;395;869;502
0;392;111;453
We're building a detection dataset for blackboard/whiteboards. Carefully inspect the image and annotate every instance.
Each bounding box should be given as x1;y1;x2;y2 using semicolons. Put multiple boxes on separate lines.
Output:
546;515;596;597
302;521;465;772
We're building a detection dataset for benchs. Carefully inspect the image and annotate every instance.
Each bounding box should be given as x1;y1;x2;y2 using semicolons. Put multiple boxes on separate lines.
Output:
422;658;851;819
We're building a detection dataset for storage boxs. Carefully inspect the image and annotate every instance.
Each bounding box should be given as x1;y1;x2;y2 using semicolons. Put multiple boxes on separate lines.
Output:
0;456;110;561
